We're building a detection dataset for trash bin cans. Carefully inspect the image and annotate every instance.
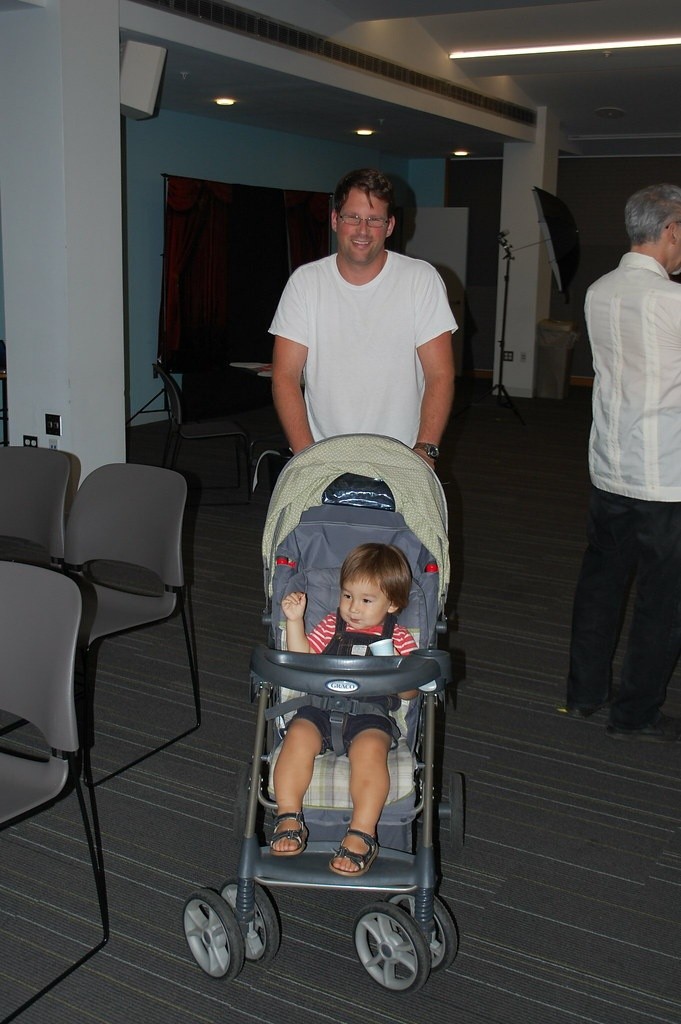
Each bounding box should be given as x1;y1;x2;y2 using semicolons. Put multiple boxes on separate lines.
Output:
534;319;577;402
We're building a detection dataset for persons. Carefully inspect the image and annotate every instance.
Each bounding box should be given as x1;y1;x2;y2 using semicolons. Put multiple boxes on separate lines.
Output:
268;166;459;472
272;542;420;875
566;181;681;744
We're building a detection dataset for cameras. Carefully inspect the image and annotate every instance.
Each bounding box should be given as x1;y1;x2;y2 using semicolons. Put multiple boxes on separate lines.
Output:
492;229;509;244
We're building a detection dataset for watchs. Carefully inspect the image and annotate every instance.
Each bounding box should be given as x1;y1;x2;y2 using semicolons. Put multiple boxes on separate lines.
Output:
412;443;441;462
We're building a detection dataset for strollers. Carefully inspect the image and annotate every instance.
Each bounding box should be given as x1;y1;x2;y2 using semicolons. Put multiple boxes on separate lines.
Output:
179;433;470;994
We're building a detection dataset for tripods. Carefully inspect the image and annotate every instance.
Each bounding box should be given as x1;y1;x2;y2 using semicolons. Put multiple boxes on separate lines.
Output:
452;249;521;420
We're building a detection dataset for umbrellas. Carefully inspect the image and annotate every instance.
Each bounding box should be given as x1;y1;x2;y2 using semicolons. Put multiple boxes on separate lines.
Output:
511;184;581;299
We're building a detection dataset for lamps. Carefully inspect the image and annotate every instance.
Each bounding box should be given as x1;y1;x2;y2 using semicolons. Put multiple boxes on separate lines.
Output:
449;35;681;61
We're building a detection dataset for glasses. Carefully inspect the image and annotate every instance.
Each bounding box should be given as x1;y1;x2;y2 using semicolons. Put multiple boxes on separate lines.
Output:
338;212;390;228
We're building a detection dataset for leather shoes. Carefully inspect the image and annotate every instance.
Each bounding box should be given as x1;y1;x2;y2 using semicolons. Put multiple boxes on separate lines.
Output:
565;684;621;718
605;709;680;744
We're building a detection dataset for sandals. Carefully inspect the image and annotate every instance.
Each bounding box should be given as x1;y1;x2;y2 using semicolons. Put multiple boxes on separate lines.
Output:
328;826;382;877
270;811;309;856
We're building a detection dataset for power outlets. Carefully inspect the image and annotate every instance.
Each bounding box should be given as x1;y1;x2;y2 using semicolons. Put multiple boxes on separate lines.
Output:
45;413;61;435
23;435;38;447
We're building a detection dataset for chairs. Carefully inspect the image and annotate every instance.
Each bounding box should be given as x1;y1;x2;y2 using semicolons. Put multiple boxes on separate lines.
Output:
0;444;81;573
148;362;252;506
60;458;206;789
0;561;112;1023
